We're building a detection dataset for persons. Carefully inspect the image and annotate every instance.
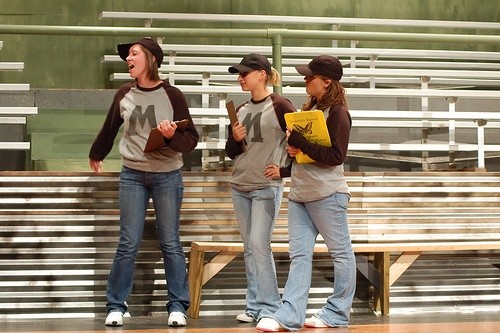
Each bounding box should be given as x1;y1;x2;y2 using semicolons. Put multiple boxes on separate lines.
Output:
256;56;357;331
225;53;299;323
88;37;199;327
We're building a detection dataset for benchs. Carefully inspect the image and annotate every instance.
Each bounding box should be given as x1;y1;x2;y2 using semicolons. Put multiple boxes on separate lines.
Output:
0;40;38;167
97;11;500;172
185;241;500;320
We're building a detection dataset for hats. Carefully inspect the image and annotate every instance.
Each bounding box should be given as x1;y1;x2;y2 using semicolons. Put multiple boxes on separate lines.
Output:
228;53;271;75
295;55;343;80
117;38;164;68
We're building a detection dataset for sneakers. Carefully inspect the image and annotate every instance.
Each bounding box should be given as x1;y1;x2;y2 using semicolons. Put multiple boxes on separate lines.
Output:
105;311;124;325
168;312;187;326
236;313;254;322
256;318;287;331
304;316;334;328
